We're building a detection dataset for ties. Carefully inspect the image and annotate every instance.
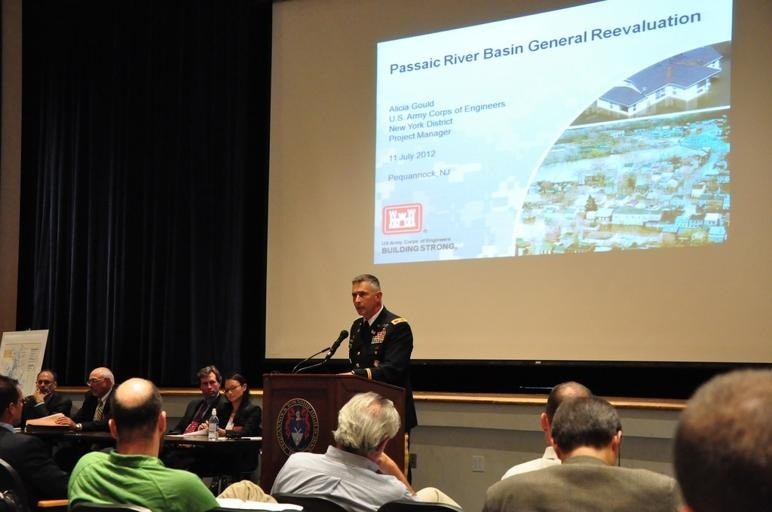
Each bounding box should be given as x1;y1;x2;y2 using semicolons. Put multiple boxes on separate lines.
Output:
362;321;369;344
96;401;103;423
184;399;207;435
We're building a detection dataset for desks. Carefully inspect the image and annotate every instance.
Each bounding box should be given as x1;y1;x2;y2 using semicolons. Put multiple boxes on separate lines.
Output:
15;430;260;496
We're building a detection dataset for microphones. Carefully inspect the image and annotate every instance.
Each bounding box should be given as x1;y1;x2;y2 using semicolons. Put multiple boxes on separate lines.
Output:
326;329;349;362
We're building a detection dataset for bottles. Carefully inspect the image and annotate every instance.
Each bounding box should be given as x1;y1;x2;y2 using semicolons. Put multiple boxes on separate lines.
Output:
208;408;220;441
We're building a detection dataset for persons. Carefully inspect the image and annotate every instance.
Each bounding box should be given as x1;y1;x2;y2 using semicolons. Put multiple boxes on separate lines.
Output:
484;368;772;512
335;274;418;486
1;366;461;511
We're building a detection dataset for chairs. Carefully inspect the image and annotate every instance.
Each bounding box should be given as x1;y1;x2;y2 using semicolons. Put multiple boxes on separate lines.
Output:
70;503;152;512
272;492;378;512
0;458;68;512
378;499;463;512
